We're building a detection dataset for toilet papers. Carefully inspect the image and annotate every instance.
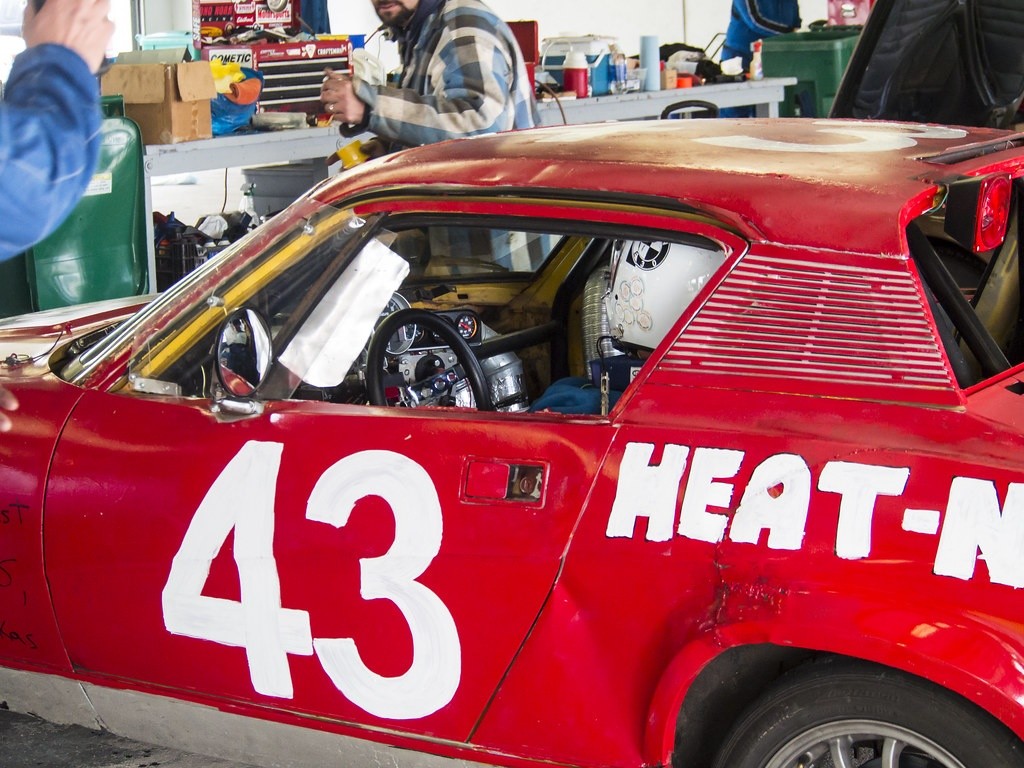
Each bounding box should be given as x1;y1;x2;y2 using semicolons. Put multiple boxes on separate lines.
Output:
639;35;661;91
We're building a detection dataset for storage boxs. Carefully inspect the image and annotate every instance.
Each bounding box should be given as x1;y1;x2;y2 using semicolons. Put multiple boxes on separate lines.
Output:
505;20;538;100
541;35;621;97
193;0;301;50
99;57;212;141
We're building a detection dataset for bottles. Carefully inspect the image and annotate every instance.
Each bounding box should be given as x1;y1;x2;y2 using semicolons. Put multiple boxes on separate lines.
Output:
750;41;764;81
640;36;661;90
609;43;629;95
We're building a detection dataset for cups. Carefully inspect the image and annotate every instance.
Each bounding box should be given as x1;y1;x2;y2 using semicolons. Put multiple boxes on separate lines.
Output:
562;50;589;98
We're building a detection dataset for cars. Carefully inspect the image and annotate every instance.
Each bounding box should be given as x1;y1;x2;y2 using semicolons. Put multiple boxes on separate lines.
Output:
0;116;1024;768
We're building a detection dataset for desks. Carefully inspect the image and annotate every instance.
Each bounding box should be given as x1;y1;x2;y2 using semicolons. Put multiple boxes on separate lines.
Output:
143;78;799;298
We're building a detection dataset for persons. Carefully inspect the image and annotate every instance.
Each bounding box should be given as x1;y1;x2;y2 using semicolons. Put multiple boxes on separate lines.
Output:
715;0;802;118
0;1;115;265
513;239;731;418
320;0;563;274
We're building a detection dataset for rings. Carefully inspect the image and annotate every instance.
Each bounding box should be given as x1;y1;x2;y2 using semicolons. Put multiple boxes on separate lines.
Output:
328;104;335;114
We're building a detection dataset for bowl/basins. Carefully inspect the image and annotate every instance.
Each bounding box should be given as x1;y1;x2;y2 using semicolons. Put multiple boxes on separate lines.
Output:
135;31;200;62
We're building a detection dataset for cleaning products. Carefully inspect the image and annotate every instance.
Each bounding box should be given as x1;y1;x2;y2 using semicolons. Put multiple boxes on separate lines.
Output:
749;42;764;81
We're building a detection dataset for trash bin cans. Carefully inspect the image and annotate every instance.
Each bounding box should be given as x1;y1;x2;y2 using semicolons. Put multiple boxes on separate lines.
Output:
750;26;863;120
0;92;151;321
241;161;330;226
